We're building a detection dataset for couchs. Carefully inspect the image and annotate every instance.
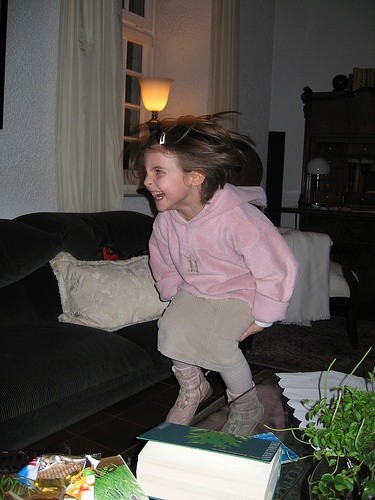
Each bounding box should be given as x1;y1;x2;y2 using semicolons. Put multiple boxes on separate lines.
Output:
0;211;176;451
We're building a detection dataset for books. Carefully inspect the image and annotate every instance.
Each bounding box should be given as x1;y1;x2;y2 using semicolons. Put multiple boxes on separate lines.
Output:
247;432;298;464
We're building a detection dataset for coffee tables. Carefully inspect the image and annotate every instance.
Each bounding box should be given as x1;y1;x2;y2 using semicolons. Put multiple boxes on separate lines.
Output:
131;374;357;500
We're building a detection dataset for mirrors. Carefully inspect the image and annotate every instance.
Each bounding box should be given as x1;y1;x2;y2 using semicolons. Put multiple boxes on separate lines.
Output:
344;142;375;194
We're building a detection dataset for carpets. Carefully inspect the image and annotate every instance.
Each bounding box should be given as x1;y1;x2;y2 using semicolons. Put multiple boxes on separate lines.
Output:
244;316;375;378
125;368;316;500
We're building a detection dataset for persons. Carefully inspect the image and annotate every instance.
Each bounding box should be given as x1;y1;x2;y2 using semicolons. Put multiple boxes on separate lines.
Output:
131;111;298;441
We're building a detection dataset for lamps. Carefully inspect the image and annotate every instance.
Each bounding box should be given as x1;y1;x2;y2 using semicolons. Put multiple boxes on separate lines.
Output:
346;146;373;194
304;156;331;208
138;77;174;134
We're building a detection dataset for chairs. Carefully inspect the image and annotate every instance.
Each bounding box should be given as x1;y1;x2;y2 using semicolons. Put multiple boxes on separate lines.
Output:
278;228;359;348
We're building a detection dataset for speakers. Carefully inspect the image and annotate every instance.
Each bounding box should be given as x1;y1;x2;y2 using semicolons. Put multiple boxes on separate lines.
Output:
264;131;285;226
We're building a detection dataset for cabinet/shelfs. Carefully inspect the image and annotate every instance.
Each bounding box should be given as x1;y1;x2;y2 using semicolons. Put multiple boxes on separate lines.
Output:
281;203;375;320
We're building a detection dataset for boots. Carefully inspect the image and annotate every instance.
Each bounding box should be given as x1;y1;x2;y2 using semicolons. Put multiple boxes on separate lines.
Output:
220;380;265;438
165;364;212;425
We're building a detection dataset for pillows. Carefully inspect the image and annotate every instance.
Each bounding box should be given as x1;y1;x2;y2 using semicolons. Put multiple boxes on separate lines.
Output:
50;250;172;332
0;220;65;289
